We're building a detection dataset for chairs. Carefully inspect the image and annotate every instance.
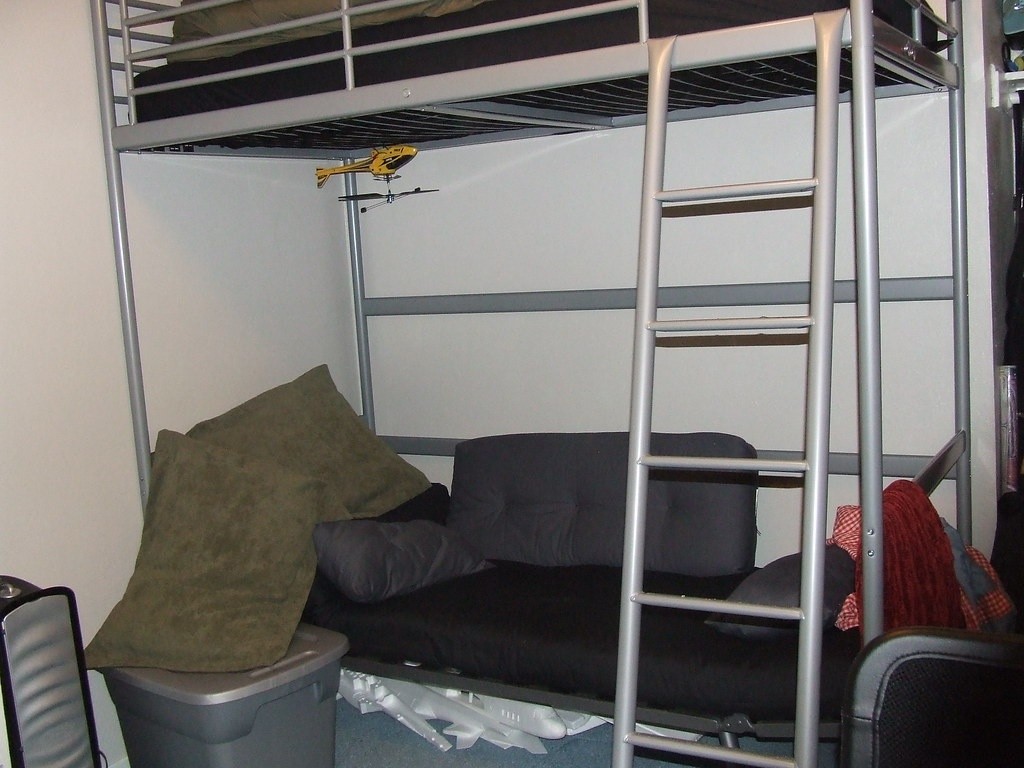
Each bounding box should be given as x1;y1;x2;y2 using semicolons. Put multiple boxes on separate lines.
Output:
836;628;1024;768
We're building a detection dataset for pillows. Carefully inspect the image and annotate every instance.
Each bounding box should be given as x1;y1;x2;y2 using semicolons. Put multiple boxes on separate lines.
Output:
82;428;326;676
445;431;760;578
705;545;855;640
315;513;499;604
190;363;433;520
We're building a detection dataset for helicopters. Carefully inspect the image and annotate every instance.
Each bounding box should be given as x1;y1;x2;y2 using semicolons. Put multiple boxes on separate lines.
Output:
315;142;440;213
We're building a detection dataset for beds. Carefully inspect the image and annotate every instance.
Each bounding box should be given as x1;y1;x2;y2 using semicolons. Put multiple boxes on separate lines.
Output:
87;0;975;768
